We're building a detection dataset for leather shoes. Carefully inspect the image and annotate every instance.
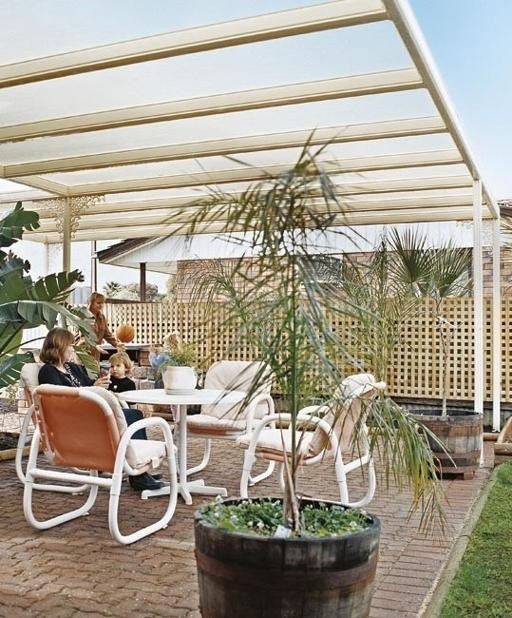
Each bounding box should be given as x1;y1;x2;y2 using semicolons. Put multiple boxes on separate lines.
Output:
129;471;164;491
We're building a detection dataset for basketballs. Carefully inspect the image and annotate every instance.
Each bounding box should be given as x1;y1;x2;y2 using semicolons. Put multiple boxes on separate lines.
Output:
116;326;135;342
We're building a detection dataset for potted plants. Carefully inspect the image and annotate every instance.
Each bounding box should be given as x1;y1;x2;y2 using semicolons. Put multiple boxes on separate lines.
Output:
413;232;484;480
152;121;406;615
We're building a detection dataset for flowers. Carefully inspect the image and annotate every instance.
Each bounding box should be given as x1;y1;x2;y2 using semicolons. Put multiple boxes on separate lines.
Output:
156;330;198;379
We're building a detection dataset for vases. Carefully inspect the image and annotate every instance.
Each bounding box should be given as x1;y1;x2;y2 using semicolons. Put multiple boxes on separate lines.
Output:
162;365;197;396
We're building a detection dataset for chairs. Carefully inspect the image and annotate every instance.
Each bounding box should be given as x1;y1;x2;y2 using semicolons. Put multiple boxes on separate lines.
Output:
234;373;377;507
23;384;178;545
15;362;130;496
174;359;276;488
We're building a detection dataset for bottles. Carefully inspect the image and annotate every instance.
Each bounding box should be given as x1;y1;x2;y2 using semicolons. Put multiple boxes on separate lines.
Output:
100;362;111;378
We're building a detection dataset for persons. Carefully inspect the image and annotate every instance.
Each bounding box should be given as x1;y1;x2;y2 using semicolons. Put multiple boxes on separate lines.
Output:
77;291;128;377
103;351;137;409
37;326;165;492
147;332;183;435
